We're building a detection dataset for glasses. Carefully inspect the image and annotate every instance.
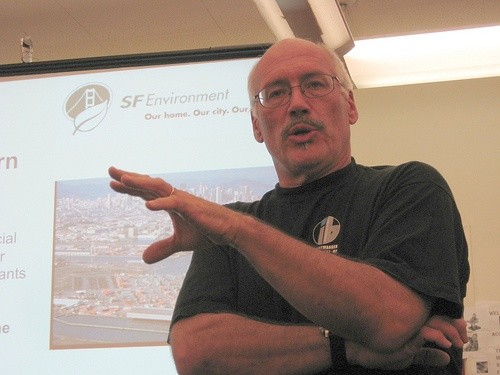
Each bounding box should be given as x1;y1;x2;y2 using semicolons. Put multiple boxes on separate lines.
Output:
254;73;349;110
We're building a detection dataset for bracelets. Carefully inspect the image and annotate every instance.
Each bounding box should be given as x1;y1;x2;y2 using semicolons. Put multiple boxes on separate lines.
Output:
319;325;350;370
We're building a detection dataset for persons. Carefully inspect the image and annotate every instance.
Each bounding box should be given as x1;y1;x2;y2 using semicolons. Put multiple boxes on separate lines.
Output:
107;36;468;374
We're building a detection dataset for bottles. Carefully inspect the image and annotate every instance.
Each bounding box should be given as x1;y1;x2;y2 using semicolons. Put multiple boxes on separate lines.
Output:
21;30;33;63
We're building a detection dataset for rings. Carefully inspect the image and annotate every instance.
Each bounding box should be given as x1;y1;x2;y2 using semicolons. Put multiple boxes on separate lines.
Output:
168;186;175;197
